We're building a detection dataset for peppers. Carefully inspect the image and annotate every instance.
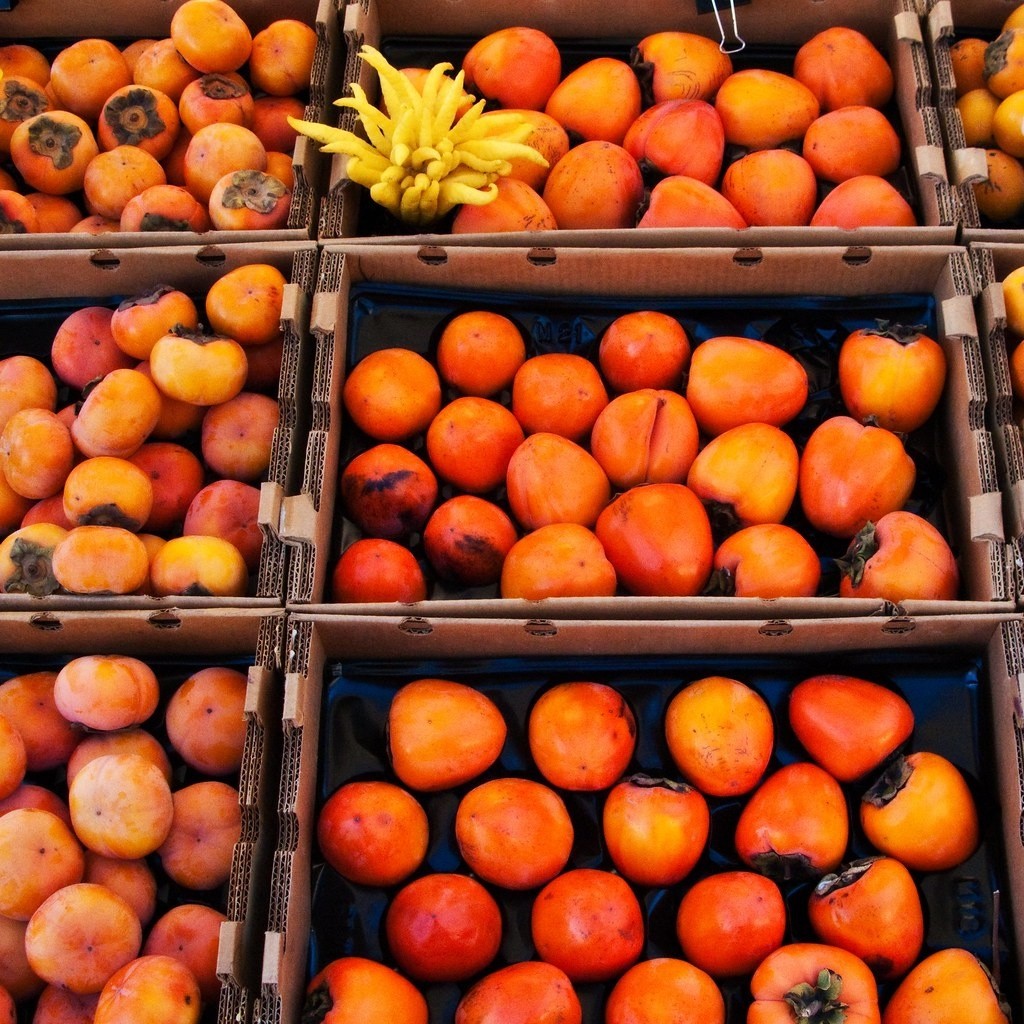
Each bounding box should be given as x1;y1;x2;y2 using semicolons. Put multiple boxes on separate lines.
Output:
285;44;550;224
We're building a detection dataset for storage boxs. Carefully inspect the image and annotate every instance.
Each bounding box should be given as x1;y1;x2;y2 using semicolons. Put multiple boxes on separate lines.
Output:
314;0;961;248
915;1;1024;248
251;608;1024;1024
0;603;287;1024
1;0;348;252
277;238;1019;618
1;247;318;613
